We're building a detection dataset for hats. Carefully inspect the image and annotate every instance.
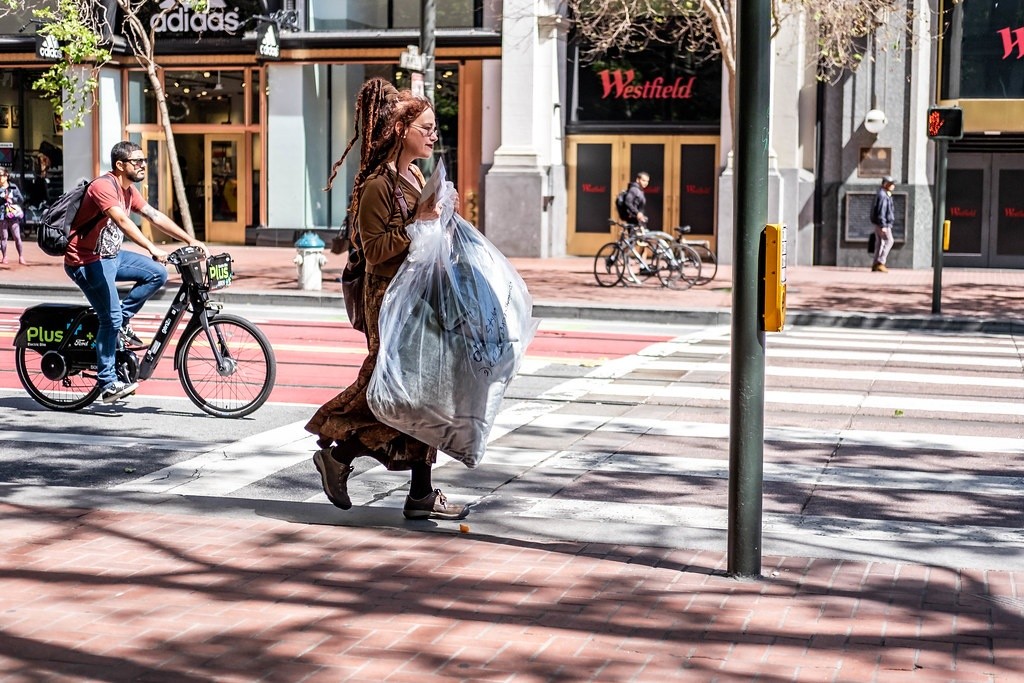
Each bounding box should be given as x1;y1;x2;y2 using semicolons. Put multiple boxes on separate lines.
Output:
883;176;896;182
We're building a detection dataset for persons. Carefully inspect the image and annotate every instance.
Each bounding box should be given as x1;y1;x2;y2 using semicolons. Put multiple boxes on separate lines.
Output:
0;166;32;266
303;78;472;521
869;175;896;273
624;170;659;275
63;141;210;403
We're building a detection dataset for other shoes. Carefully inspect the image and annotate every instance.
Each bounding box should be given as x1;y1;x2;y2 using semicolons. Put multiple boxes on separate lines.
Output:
605;256;614;274
872;265;879;271
874;263;888;272
640;266;652;274
3;258;8;264
19;258;25;265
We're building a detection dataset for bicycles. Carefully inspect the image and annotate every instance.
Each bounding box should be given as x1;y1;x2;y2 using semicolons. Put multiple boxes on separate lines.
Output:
593;219;719;292
13;246;277;419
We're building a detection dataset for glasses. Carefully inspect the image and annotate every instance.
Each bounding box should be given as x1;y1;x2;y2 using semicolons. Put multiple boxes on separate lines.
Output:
121;158;147;165
410;122;438;136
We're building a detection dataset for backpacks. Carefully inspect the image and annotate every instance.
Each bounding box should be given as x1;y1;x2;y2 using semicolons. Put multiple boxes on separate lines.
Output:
37;175;118;256
616;191;630;221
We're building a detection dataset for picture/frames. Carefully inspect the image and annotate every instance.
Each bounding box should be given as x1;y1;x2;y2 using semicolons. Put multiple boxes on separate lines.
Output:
0;105;9;127
11;105;19;128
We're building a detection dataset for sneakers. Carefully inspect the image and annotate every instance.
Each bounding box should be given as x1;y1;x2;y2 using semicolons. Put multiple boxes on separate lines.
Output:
102;381;139;403
313;446;354;510
119;324;143;346
403;489;469;520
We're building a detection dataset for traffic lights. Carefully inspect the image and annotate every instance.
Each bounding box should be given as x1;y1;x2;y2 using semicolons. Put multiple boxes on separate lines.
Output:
926;105;965;140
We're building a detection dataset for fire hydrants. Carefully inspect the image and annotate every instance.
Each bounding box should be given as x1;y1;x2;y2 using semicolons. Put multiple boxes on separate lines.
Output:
293;229;328;292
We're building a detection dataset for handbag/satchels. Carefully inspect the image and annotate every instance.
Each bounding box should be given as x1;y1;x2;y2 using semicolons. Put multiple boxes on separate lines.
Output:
5;204;24;221
868;233;876;252
342;249;367;332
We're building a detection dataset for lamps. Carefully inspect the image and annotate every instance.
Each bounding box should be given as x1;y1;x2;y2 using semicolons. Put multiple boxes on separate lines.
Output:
174;79;182;88
17;19;48;33
